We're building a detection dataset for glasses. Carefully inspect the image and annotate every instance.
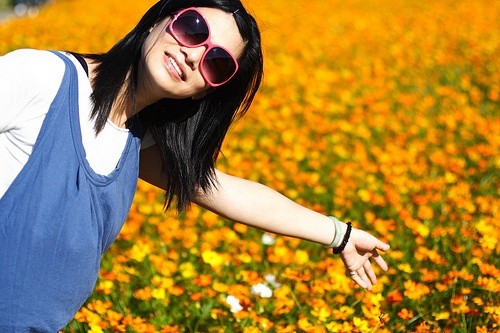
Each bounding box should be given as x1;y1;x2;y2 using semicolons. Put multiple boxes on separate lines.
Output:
168;7;239;87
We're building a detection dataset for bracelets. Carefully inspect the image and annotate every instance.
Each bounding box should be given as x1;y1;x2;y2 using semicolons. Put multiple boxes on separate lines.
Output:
322;214;342;248
332;221;353;255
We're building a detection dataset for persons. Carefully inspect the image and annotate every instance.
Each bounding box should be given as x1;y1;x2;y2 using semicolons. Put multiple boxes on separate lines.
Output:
0;0;391;333
